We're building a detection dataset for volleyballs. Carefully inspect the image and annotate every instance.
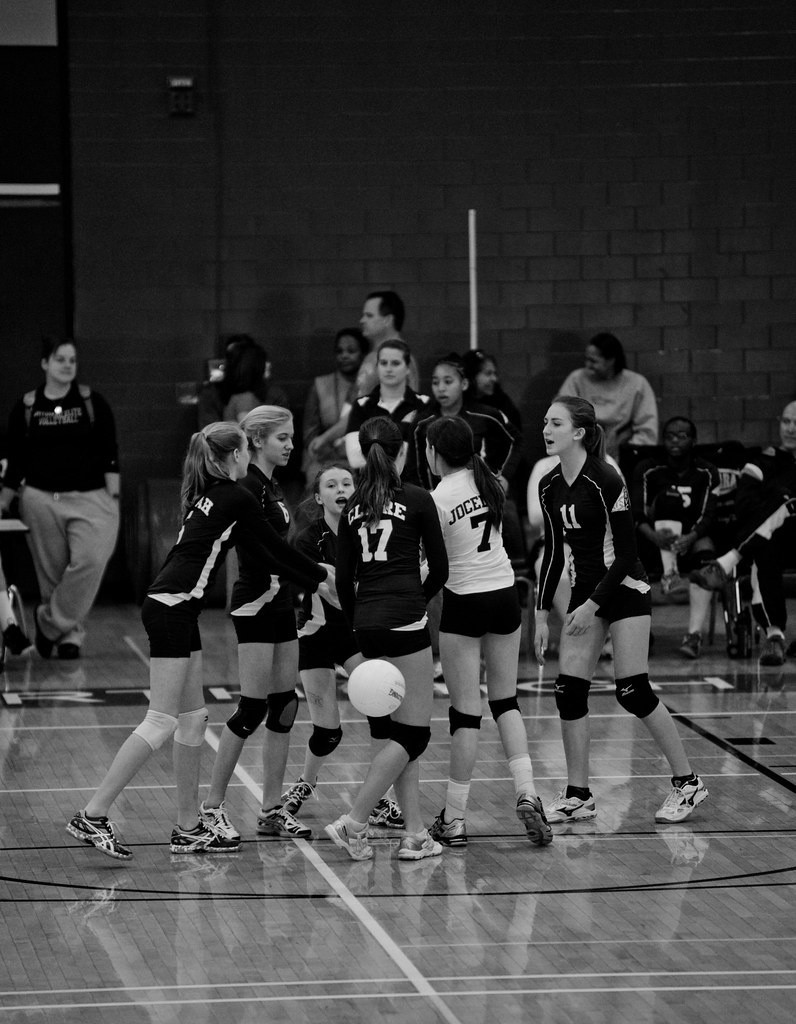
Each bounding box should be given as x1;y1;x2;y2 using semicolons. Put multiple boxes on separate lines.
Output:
346;659;406;717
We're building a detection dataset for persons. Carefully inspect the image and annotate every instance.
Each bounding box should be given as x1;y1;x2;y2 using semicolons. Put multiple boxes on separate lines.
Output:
66;292;796;862
0;337;121;673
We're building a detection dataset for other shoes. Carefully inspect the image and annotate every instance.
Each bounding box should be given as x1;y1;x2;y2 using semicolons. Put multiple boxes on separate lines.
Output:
3;623;35;659
600;633;615;659
432;660;442;679
688;559;732;591
680;631;701;658
760;635;786;665
57;643;80;660
335;666;349;679
33;603;54;659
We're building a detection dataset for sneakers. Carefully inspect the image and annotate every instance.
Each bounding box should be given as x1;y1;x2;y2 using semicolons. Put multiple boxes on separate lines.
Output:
517;794;553;845
654;775;709;822
198;801;241;841
398;829;443;859
544;785;597;823
428;808;467;846
368;797;404;828
281;775;318;813
324;815;374;861
68;825;709;926
256;800;312;837
169;820;244;854
65;808;133;861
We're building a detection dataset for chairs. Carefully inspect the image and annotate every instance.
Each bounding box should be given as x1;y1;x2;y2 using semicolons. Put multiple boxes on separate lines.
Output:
516;440;796;660
